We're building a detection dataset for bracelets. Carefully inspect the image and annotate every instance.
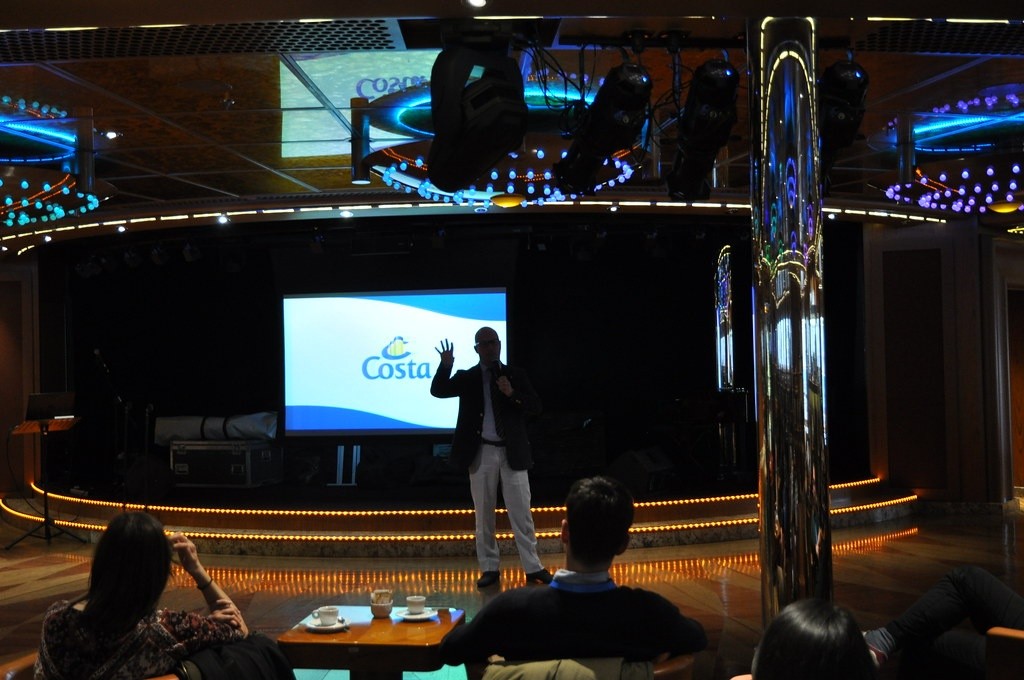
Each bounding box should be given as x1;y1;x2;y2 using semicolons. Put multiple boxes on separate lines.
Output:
197;577;212;590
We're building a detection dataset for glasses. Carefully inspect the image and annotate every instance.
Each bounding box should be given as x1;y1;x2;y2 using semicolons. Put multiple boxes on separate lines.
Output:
476;339;499;348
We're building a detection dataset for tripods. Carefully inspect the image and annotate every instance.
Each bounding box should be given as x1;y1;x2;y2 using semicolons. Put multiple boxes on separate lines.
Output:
6;417;87;549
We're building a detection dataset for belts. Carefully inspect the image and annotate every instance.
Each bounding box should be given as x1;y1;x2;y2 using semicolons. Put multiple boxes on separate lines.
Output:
483;439;506;447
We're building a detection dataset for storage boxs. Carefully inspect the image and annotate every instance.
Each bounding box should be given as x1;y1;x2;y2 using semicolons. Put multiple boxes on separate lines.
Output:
170;439;284;488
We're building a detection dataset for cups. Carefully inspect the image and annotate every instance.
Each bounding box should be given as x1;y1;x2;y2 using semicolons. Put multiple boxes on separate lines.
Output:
370;599;394;618
406;596;426;614
312;606;340;626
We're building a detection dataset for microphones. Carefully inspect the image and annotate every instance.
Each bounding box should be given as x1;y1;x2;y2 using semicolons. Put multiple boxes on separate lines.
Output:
491;360;502;381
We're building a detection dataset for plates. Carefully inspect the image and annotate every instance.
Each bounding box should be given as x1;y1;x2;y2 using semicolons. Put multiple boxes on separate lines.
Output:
397;609;438;620
306;616;351;631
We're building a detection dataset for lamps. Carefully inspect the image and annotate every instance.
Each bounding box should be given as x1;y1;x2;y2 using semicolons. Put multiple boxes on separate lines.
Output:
816;48;870;171
550;43;653;196
428;27;529;192
665;47;740;201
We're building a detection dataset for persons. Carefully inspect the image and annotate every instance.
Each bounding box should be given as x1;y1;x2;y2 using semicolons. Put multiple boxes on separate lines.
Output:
430;326;553;589
33;511;250;680
860;566;1024;680
751;597;879;680
440;477;707;665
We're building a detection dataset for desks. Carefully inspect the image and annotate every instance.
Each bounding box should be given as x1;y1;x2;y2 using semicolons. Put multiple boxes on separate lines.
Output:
277;605;466;680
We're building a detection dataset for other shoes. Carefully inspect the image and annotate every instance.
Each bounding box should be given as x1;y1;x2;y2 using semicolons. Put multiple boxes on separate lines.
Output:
477;570;500;587
526;568;553;584
861;630;888;672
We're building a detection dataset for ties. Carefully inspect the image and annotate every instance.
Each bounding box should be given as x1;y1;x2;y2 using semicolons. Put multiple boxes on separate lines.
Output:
488;367;506;439
